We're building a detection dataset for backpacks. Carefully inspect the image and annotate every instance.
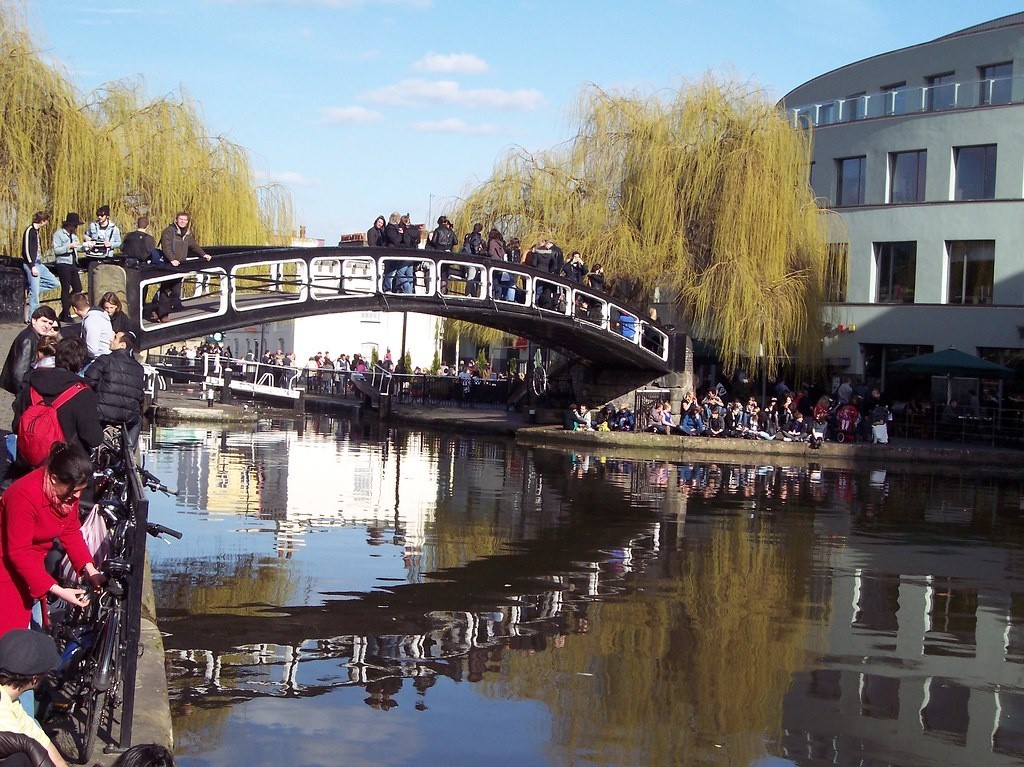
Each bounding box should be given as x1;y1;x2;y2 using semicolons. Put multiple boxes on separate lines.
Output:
19;382;91;469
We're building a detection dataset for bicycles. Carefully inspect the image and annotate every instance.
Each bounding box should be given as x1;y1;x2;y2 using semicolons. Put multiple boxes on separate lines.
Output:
64;421;185;763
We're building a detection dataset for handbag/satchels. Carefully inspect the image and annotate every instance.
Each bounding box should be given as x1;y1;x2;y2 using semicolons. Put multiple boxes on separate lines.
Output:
86;240;109;258
151;248;174;271
58;504;112;584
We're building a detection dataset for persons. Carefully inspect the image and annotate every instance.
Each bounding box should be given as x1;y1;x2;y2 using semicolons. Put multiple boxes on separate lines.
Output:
574;404;592;431
82;205;122;311
612;403;635;433
110;743;175;767
0;730;57;767
0;627;67;767
164;340;546;415
0;290;157;456
365;212;676;357
150;212;212;323
20;209;60;324
562;403;588;431
1;440;108;638
644;369;1024;450
52;212;83;323
591;402;618;432
1;334;105;491
119;216;156;309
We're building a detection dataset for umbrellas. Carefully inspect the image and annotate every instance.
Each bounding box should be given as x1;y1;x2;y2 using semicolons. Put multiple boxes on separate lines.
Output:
886;343;1018;407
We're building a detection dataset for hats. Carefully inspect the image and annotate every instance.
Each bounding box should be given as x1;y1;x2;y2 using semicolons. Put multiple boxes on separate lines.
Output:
100;205;109;214
62;213;85;225
0;628;59;676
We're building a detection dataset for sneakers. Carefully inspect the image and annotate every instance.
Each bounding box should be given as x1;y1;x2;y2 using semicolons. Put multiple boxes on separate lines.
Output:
45;597;69;612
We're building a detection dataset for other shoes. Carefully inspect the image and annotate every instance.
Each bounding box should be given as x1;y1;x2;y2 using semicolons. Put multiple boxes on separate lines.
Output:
59;311;76;323
152;312;170;322
